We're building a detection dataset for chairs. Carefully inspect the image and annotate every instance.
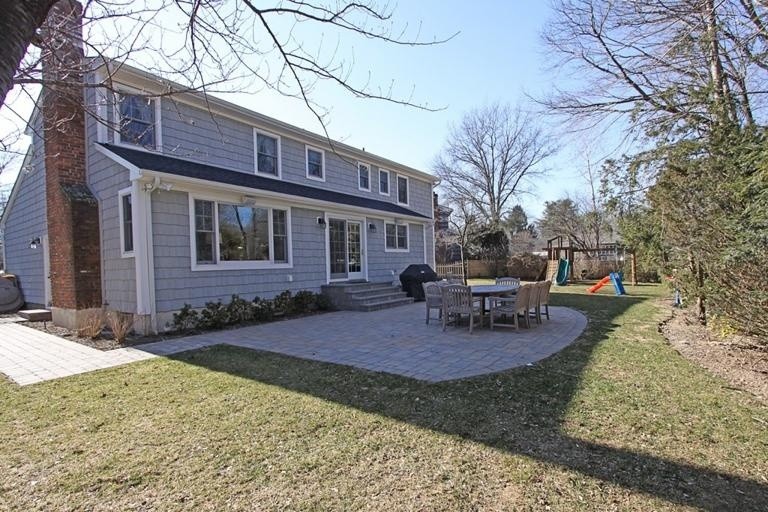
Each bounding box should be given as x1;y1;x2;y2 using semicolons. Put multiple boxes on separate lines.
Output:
421;272;552;334
